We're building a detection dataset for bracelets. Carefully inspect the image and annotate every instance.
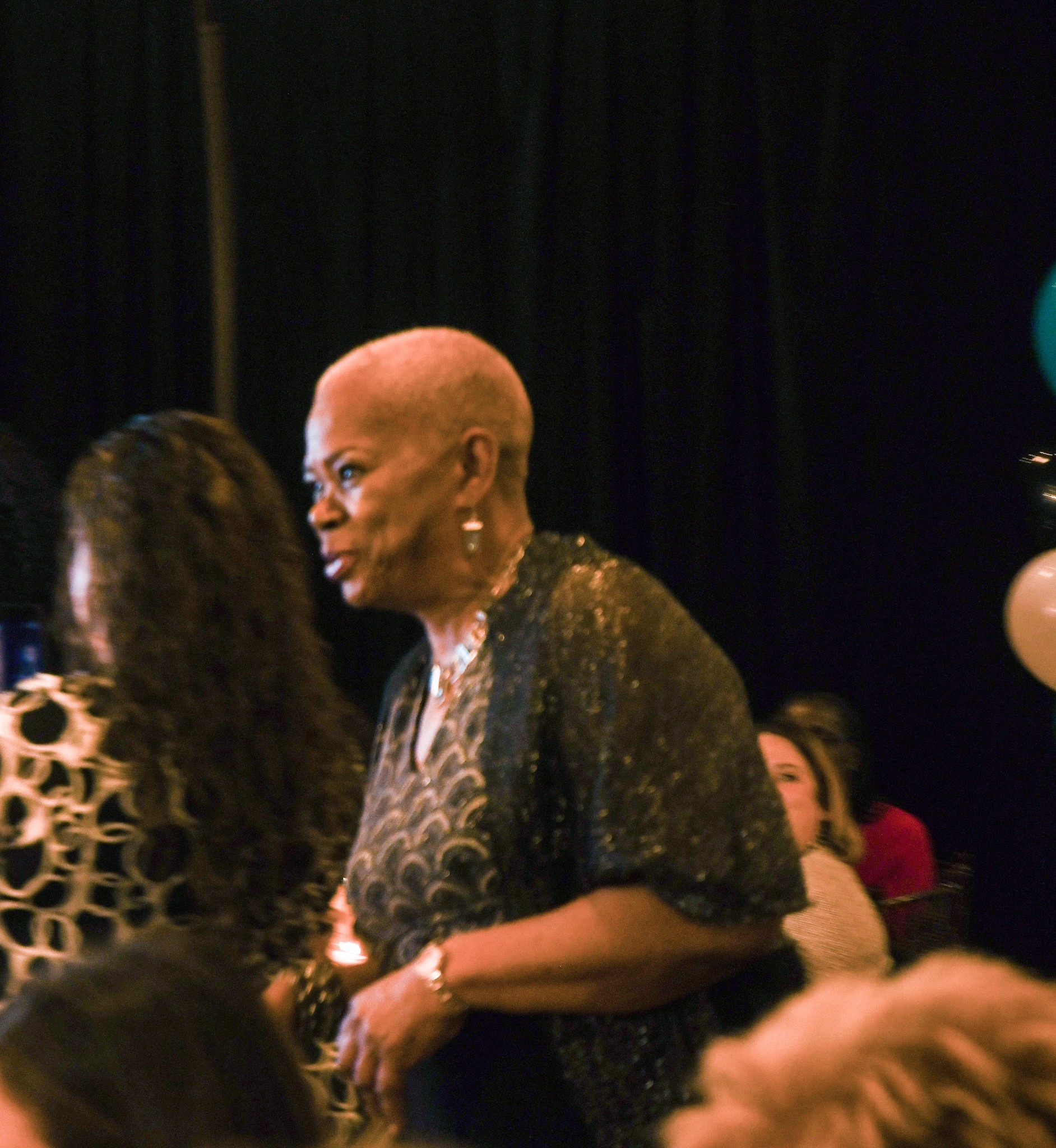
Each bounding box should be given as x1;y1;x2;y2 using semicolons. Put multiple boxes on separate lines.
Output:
282;959;346;1030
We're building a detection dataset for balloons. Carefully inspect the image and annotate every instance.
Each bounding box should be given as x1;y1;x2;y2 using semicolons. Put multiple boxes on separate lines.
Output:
1036;268;1056;392
1006;550;1056;689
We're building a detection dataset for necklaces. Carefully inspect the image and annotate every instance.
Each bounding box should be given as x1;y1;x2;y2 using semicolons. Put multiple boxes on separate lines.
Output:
431;546;524;697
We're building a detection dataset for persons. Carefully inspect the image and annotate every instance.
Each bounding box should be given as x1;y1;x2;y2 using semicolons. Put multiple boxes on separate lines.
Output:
1;937;320;1148
755;700;928;978
260;329;803;1148
1;411;366;997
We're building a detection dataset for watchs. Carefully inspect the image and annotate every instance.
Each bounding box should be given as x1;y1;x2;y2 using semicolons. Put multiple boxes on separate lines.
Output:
416;943;469;1020
